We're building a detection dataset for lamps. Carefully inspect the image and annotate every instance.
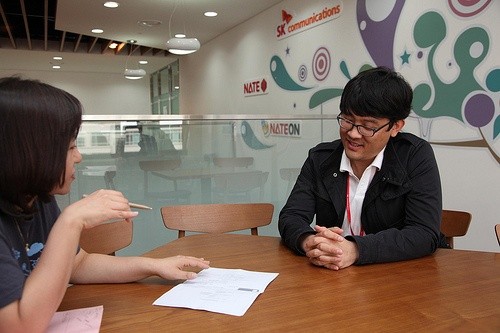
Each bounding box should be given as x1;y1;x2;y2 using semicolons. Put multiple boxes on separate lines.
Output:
166;37;201;56
124;68;146;80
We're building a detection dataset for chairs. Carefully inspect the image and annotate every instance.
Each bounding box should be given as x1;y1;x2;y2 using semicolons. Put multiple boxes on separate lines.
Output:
160;203;274;238
213;157;253;199
140;159;193;204
80;221;133;256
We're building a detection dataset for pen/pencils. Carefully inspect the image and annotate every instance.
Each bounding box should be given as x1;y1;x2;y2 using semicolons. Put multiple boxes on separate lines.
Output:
82;194;152;211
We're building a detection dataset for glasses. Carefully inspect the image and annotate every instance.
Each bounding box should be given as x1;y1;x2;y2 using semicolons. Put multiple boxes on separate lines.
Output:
337;116;394;137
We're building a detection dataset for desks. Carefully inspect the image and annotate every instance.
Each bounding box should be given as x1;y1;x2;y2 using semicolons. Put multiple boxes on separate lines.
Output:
56;232;500;333
152;167;250;203
